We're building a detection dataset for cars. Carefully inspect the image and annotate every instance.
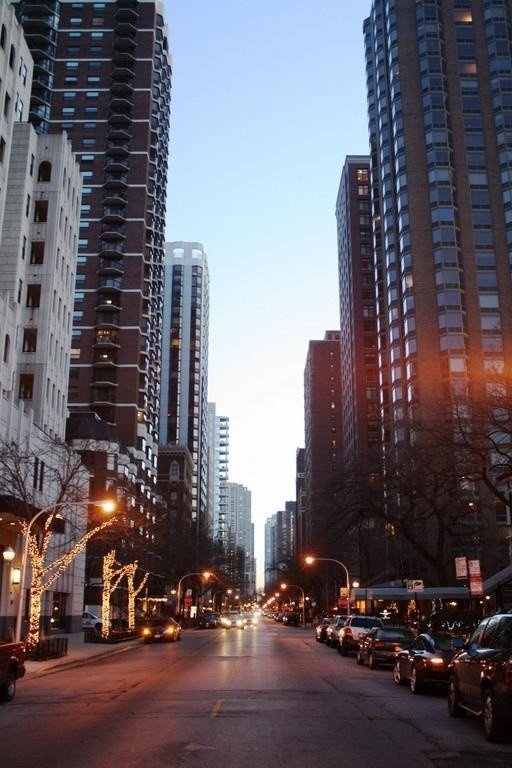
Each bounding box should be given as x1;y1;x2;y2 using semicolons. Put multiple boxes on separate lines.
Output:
268;612;299;626
199;612;256;630
82;612;113;631
144;617;181;643
315;614;512;740
0;641;26;700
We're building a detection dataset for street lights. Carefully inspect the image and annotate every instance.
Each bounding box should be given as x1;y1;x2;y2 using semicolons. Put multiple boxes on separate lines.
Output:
178;572;213;616
305;556;350;614
281;584;306;627
15;499;116;643
